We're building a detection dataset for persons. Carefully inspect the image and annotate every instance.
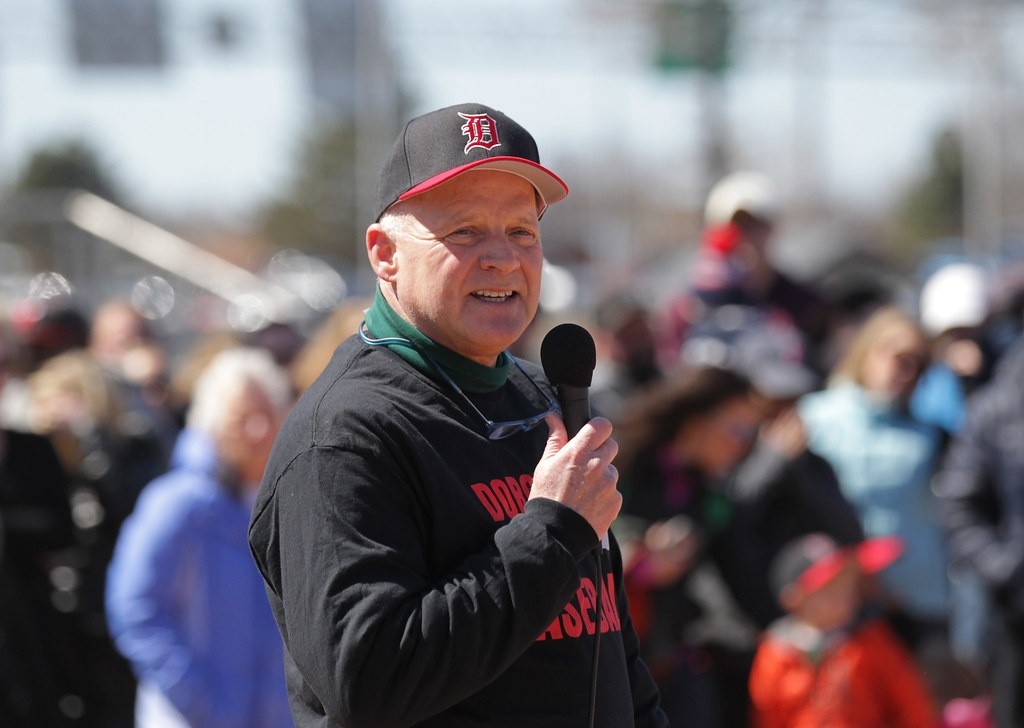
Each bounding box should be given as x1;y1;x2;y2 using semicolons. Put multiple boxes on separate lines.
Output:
0;166;1024;728
246;103;672;728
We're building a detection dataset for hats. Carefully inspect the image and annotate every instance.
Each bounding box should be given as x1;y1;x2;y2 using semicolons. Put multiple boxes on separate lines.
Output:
917;263;994;337
704;171;774;233
374;103;569;226
772;532;905;607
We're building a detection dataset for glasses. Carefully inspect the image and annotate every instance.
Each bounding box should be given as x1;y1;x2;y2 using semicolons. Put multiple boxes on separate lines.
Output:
359;313;559;442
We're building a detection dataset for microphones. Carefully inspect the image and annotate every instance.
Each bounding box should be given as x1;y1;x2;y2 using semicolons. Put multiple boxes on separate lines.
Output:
540;323;604;560
